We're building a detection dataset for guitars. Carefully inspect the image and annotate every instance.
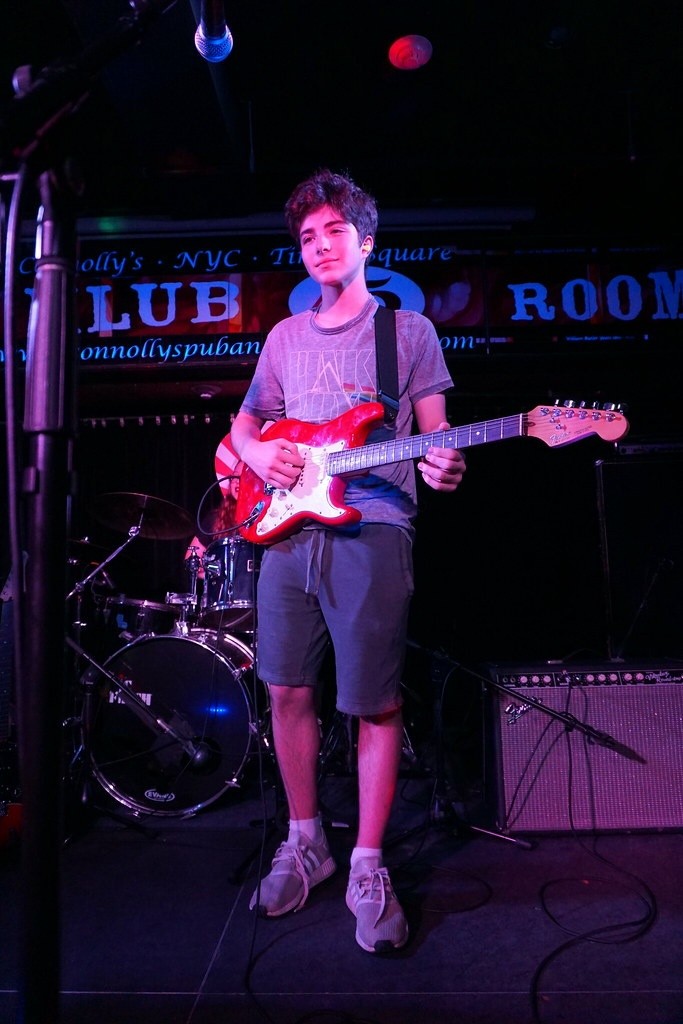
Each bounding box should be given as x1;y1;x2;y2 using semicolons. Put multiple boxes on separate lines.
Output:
234;397;632;545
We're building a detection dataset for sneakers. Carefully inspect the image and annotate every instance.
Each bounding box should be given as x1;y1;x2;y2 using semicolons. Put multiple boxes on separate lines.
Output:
250;812;338;917
346;856;409;953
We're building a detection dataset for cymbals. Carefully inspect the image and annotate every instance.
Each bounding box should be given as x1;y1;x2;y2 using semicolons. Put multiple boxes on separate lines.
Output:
82;491;198;541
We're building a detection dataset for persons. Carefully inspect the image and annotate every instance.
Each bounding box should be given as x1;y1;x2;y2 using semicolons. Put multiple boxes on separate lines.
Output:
186;459;245;579
229;171;466;952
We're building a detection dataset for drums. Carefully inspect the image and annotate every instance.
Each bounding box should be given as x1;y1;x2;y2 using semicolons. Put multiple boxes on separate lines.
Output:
165;590;200;632
91;594;181;659
80;626;272;820
199;536;264;630
